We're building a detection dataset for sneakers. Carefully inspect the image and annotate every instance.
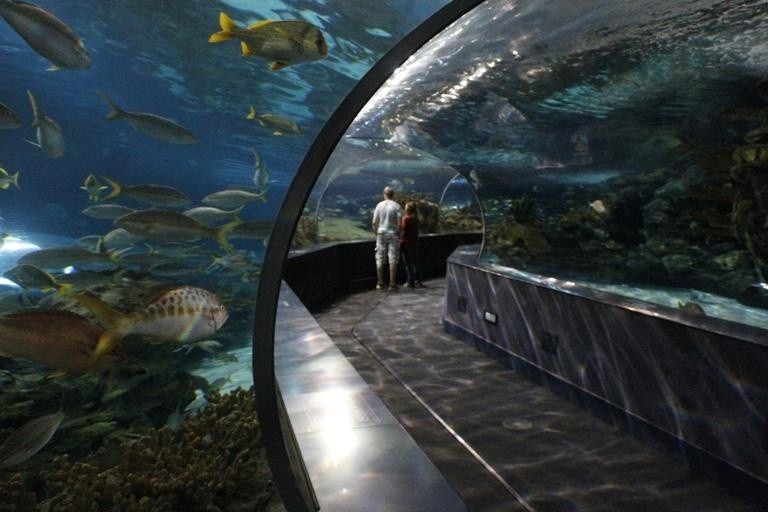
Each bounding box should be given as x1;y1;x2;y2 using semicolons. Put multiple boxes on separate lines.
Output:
376;282;384;290
401;282;414;288
415;282;422;288
388;285;400;291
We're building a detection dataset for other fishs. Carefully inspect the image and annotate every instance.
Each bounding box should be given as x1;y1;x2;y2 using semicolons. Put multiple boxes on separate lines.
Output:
0;174;272;470
207;11;328;72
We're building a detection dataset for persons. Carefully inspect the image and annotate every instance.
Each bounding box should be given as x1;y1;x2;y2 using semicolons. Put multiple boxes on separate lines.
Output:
370;186;404;292
399;201;425;288
